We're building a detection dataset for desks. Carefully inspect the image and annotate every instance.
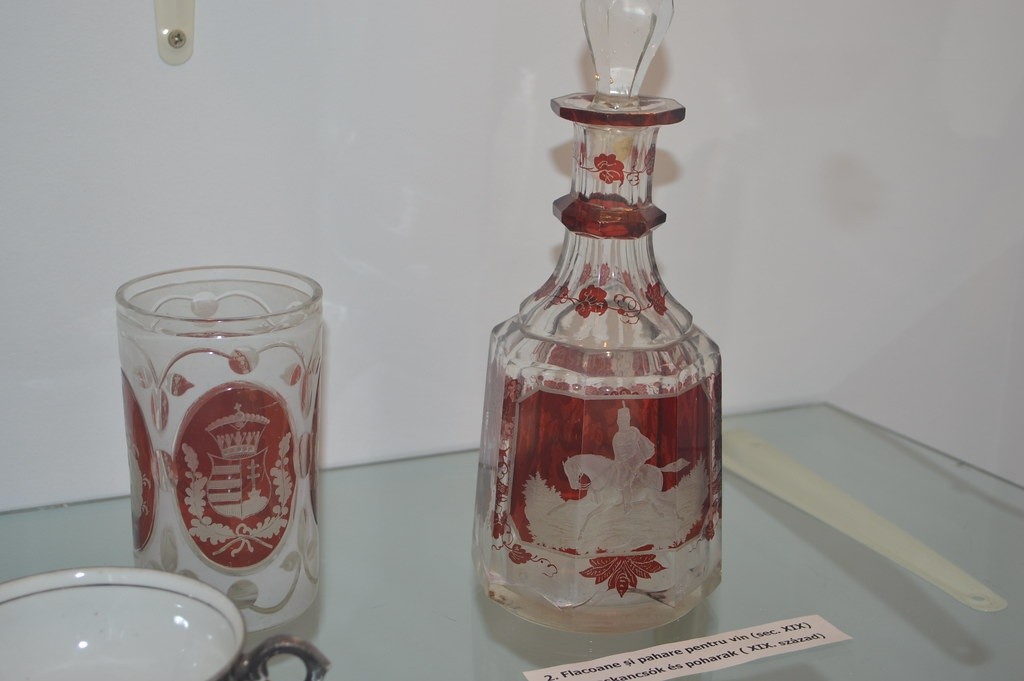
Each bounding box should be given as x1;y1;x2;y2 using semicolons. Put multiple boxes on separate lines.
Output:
0;403;1024;681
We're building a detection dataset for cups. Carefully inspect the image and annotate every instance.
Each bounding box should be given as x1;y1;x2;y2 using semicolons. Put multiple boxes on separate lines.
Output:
116;266;329;666
0;565;331;681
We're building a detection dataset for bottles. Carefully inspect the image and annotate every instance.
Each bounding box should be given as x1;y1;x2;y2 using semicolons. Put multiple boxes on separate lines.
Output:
471;0;721;633
